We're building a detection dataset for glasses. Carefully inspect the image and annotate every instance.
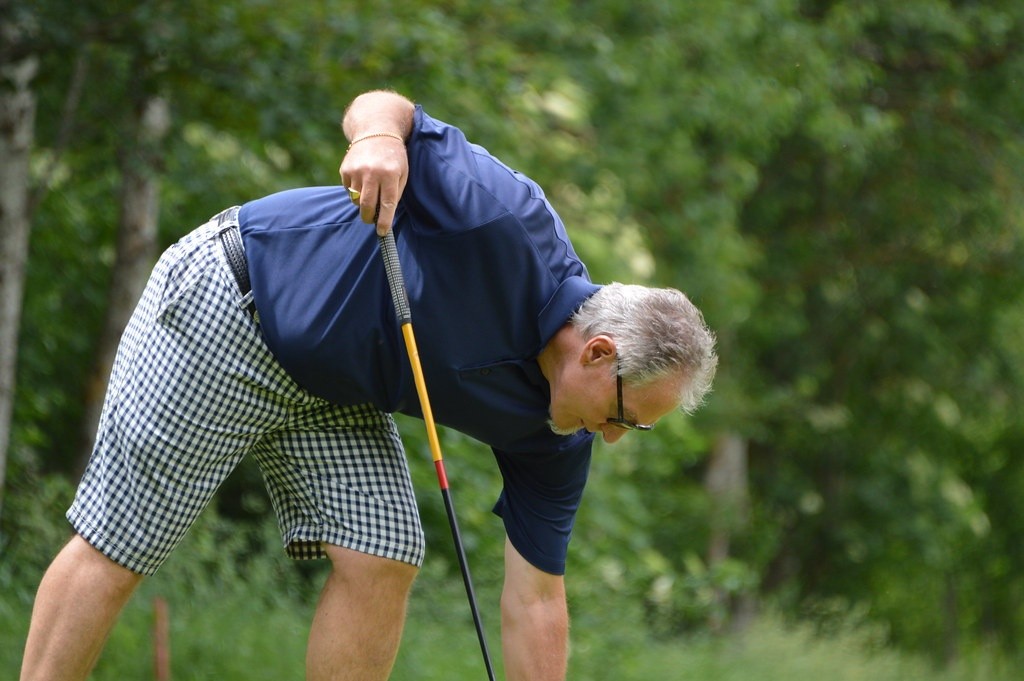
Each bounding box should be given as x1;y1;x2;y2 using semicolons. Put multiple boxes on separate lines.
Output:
605;359;656;430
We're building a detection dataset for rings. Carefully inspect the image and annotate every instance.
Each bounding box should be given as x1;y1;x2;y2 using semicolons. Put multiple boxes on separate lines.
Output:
348;187;360;200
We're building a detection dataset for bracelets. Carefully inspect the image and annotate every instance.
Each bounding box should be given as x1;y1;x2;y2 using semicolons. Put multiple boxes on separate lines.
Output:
347;132;404;152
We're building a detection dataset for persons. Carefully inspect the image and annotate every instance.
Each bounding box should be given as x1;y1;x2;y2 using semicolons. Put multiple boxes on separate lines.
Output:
17;89;721;681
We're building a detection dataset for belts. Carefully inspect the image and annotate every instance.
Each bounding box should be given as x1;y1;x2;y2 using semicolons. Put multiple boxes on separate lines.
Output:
218;208;256;318
